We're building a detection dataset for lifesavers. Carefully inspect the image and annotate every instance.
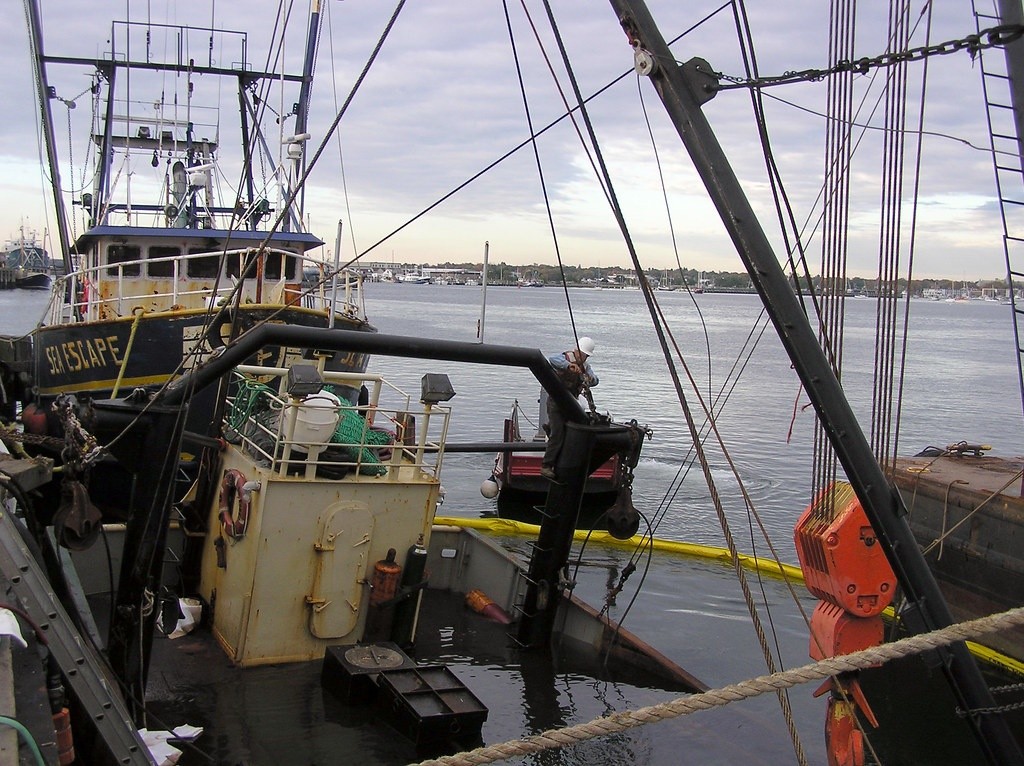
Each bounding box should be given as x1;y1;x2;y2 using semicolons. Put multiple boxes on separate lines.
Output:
218;468;251;540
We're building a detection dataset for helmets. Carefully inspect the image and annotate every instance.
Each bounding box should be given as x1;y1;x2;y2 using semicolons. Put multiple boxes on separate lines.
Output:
575;337;595;356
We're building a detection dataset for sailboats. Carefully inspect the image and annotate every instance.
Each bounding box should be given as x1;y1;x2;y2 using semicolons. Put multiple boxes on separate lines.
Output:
657;267;677;291
22;1;405;401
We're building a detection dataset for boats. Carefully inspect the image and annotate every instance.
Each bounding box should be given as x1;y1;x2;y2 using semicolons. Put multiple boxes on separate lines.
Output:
0;0;1023;765
497;401;642;506
395;272;431;284
877;446;1024;665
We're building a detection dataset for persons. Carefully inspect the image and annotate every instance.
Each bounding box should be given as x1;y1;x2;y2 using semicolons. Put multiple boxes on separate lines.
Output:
540;337;599;477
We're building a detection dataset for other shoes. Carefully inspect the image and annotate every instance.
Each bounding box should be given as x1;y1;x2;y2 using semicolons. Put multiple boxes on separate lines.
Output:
540;466;555;478
